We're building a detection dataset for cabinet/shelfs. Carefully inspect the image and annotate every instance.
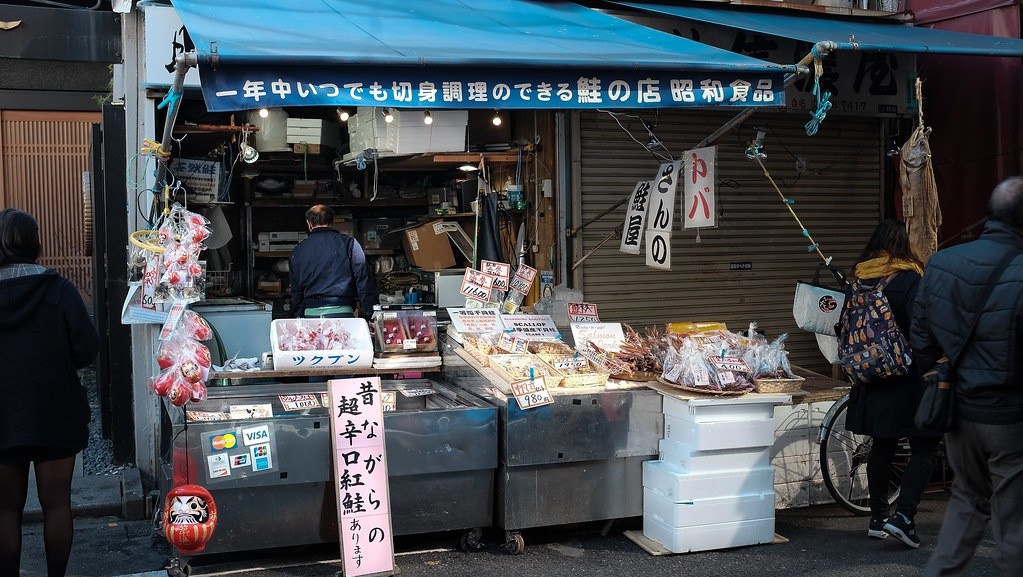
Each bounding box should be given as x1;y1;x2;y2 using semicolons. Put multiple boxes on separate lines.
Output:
240;149;538;297
775;361;871;507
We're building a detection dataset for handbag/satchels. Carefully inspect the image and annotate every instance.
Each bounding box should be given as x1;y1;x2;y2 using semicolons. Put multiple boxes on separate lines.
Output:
915;354;957;432
793;263;845;336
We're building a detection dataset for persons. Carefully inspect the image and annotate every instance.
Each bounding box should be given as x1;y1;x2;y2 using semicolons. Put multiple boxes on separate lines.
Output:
910;176;1023;577
288;205;371;383
838;220;945;548
0;208;98;577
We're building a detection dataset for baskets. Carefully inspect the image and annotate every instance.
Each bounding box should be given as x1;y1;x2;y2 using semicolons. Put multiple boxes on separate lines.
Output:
754;373;806;394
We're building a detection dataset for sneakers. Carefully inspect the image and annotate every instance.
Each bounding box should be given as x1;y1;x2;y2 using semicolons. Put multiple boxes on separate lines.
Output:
868;513;891;539
883;511;921;547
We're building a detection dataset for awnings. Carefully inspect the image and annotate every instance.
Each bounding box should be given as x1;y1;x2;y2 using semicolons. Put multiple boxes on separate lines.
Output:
606;0;1023;56
168;0;808;114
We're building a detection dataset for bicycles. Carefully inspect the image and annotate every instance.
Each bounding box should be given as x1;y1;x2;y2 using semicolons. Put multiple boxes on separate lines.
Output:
816;389;946;515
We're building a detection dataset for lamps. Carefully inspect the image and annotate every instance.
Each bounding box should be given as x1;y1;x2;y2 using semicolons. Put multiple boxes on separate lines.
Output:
260;109;271;118
335;106;349;121
491;109;502;126
241;142;259;164
381;107;393;123
423;108;433;124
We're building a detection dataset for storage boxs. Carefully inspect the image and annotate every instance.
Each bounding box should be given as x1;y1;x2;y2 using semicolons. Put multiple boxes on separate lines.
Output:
642;460;775;502
287;135;340;146
376;317;408;351
294;179;316;189
333;221;357;239
401;220;457;268
287;127;340;138
293;190;315;198
658;440;770;473
361;218;406;248
362;232;381;249
347;106;468;160
269;318;374;368
421;271;466;306
664;415;775;452
294;144;329;154
643;512;775;553
643;486;776;527
258;279;283;290
286;117;339;130
449;324;606;388
404;318;436;350
662;395;775;423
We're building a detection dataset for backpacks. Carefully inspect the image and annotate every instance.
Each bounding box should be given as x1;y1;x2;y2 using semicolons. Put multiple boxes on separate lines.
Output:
834;271;913;386
